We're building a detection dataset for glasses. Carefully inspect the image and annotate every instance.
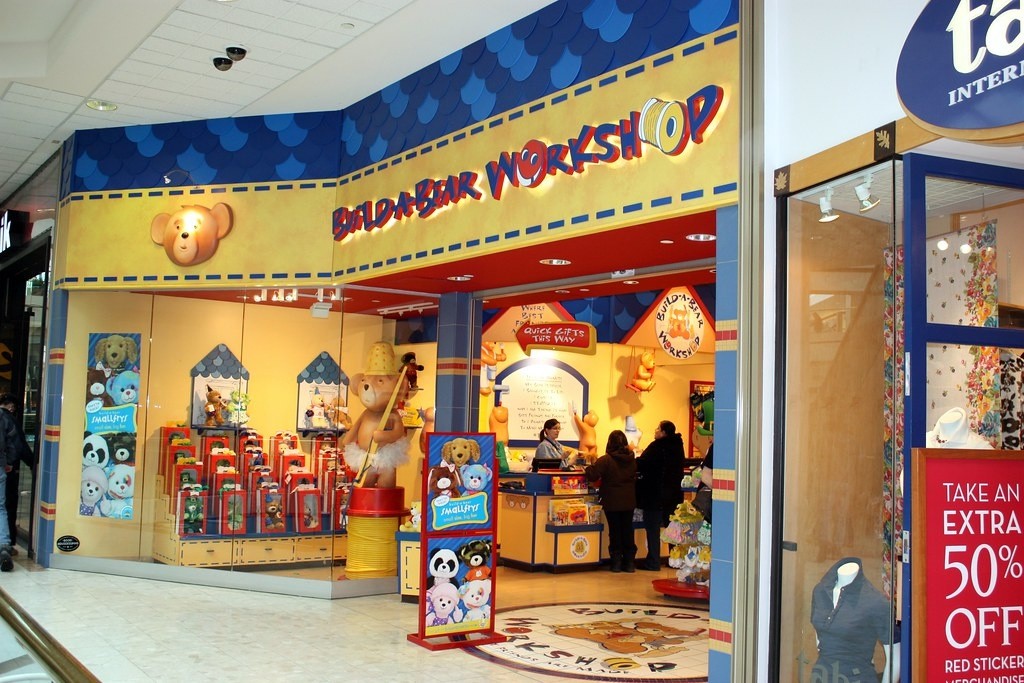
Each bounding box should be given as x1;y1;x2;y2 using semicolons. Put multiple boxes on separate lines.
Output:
551;426;560;431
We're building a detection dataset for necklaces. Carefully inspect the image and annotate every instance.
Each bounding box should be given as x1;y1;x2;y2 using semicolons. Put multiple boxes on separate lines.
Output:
936;433;949;444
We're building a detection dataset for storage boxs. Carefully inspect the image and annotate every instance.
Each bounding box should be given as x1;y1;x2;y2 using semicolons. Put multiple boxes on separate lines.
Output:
157;426;355;538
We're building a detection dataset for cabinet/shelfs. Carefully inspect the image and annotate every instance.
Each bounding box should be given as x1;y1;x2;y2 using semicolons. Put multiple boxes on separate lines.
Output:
150;474;348;568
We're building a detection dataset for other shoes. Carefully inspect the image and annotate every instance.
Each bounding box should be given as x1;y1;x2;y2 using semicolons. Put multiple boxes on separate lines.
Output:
0;544;20;569
638;559;661;571
610;561;636;573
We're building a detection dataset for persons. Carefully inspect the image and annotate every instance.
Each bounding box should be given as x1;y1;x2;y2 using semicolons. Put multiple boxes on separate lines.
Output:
0;392;38;555
810;559;901;683
0;416;15;572
585;430;639;572
899;408;996;498
535;419;578;468
635;420;685;570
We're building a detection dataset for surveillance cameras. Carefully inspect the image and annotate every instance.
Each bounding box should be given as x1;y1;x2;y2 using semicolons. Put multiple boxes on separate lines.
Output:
212;55;233;71
225;43;249;62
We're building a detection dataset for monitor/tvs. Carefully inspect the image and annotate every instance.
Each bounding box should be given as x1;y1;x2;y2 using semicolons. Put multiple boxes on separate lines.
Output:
532;457;561;472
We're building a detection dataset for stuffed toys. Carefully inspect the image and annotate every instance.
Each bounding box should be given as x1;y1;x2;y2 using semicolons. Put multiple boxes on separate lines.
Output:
632;352;656;392
204;384;250;428
244;441;284;530
480;342;507;395
399;352;424;388
278;439;317;528
170;433;203;533
211;440;243;531
342;342;410;487
428;438;493;497
426;539;492;627
320;441;349;525
81;335;139;519
306;386;353;430
